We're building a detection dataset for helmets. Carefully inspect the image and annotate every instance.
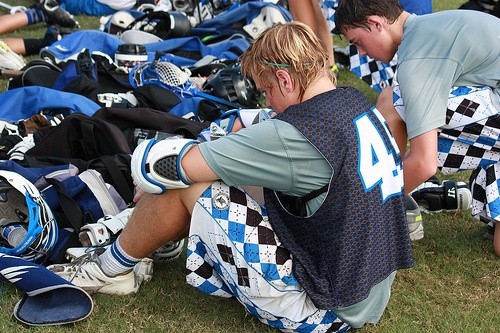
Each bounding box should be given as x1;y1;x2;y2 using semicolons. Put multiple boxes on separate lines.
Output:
99;0;214;71
201;66;259;106
128;59;192;93
0;169;59;260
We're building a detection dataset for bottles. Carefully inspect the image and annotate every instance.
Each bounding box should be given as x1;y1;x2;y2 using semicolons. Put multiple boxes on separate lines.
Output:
0;218;28;248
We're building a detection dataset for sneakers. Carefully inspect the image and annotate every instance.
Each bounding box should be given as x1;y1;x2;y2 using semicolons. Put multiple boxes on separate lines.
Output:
44;23;71;42
30;0;79;29
405;193;424;240
65;247;156;281
45;260;139;295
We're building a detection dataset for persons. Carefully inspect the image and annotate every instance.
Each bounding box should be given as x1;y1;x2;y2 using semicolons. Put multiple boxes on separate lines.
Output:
46;21;416;333
0;0;500;255
